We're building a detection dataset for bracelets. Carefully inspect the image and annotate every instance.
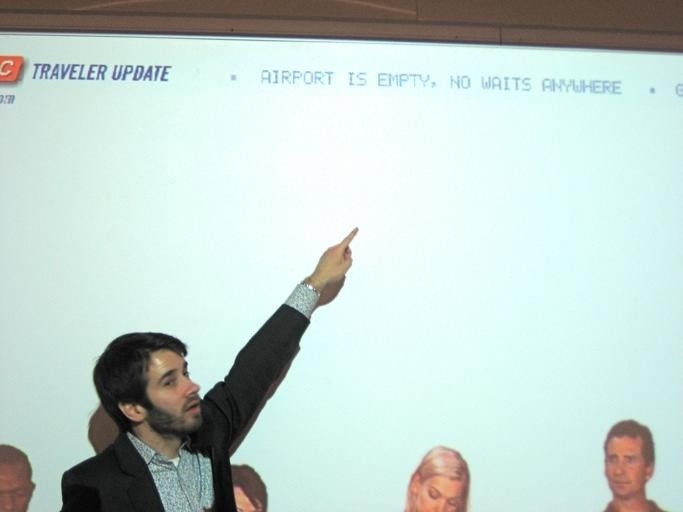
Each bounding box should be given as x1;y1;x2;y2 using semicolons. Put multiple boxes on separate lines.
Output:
301;279;319;295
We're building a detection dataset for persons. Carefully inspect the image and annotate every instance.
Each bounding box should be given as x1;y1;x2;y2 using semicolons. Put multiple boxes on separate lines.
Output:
0;443;35;511
405;444;471;511
602;419;666;511
60;226;360;512
230;463;267;511
